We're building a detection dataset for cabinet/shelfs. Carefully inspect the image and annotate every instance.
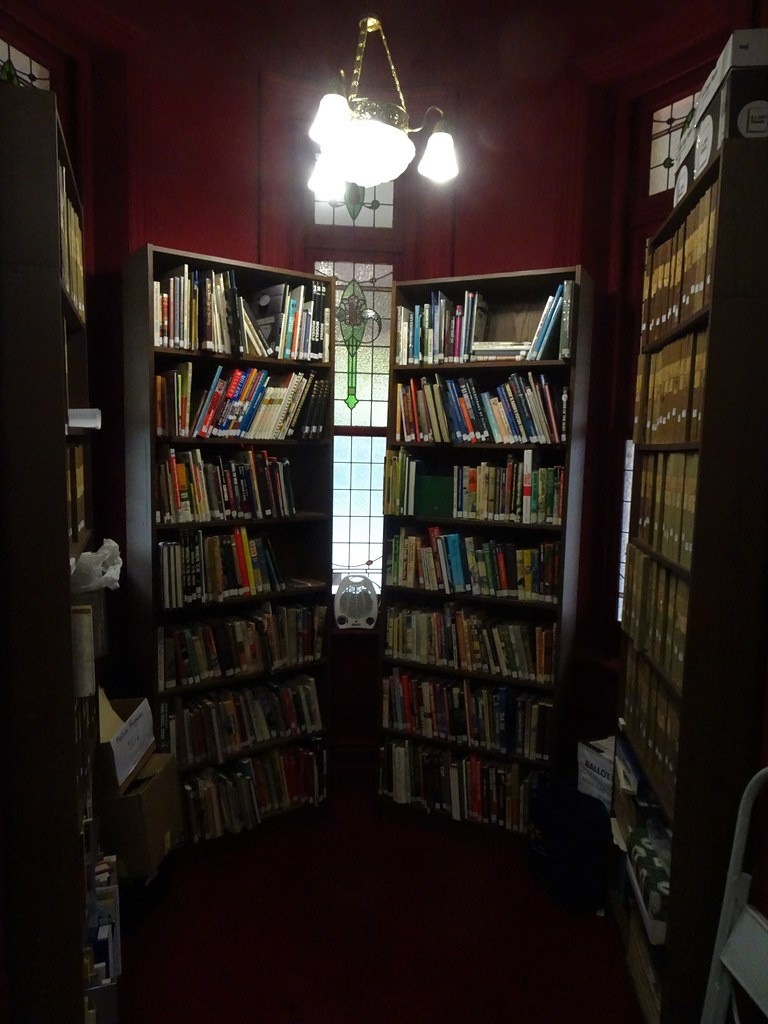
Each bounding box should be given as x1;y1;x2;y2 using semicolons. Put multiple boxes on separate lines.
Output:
0;32;768;1024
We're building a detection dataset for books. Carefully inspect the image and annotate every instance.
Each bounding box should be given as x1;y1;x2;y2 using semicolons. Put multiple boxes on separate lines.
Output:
54;168;721;1024
